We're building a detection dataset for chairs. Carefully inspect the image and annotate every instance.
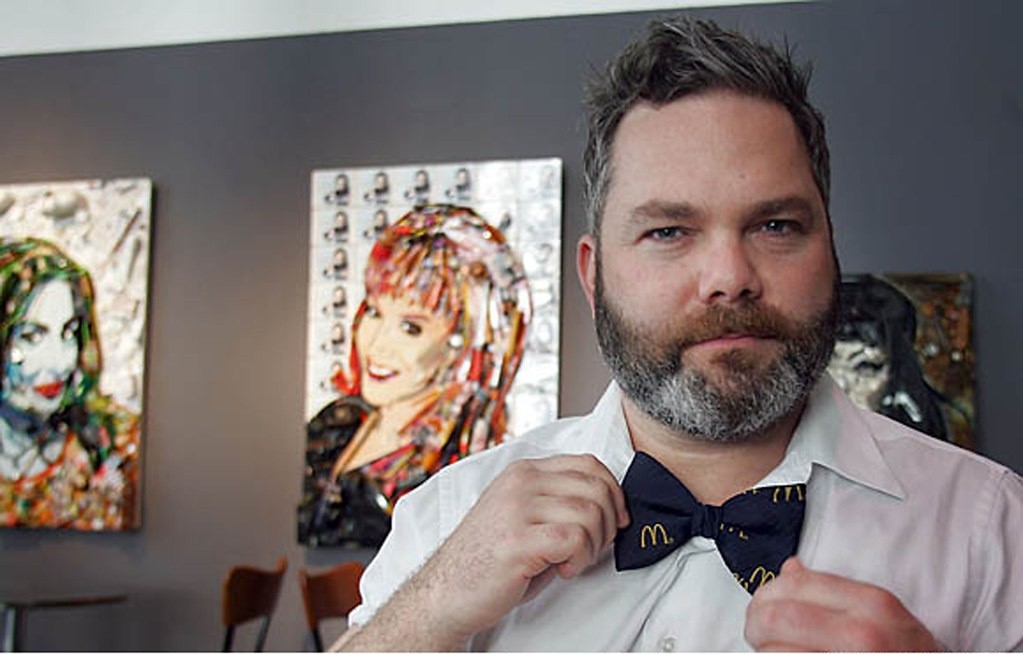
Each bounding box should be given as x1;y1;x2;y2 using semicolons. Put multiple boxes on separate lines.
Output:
216;557;290;654
298;563;370;652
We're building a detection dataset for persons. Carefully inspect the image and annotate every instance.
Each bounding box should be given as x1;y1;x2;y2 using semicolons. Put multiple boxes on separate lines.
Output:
322;18;1021;651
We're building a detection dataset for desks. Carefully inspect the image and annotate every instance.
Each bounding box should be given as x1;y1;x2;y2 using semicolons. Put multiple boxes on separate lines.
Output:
0;592;127;652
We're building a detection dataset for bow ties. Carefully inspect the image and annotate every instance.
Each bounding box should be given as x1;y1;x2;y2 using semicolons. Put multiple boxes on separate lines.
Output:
611;455;807;594
